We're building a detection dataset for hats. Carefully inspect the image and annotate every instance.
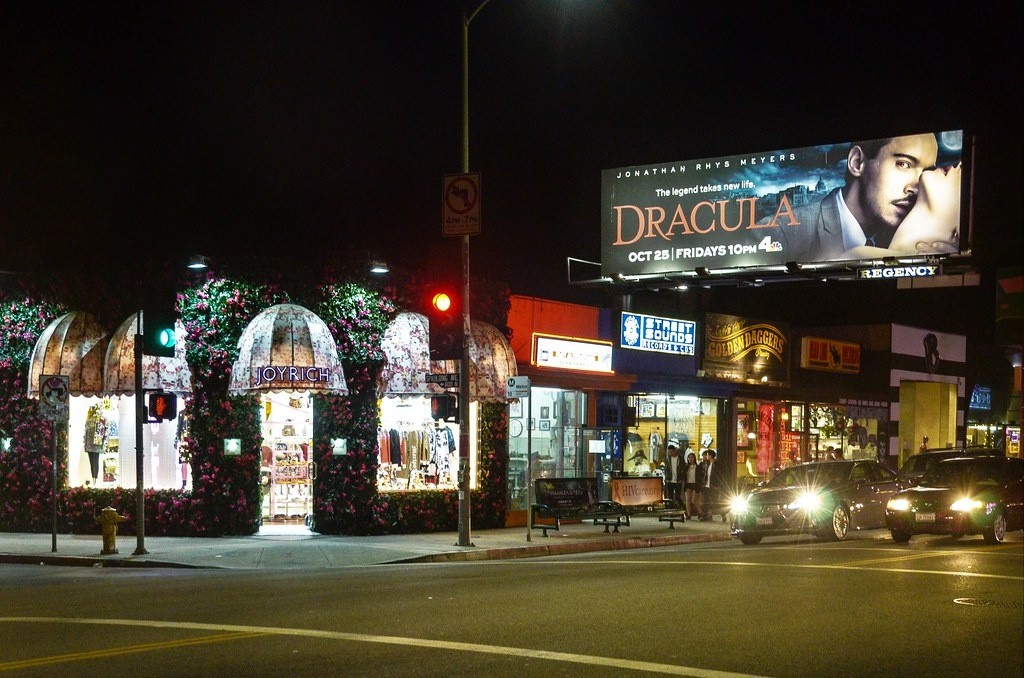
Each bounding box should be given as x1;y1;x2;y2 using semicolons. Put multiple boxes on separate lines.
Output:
628;449;648;461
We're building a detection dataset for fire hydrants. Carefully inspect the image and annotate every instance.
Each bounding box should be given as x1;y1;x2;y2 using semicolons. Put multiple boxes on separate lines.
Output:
95;505;128;553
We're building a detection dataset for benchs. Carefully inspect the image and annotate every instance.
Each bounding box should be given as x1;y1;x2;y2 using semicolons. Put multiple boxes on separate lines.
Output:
604;475;687;533
529;477;631;538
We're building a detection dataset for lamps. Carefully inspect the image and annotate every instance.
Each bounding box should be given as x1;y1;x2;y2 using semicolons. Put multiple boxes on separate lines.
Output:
367;259;390;273
186;255;210;269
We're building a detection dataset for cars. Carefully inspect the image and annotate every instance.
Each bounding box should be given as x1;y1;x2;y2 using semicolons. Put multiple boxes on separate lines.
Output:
729;460;898;542
886;458;1024;545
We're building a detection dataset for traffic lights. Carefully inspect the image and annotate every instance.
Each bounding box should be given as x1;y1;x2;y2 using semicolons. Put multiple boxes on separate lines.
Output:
145;271;177;357
431;394;452;420
429;289;464;360
150;391;180;423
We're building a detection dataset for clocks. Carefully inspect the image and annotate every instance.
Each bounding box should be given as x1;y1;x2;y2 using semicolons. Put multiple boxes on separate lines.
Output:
509;419;523;437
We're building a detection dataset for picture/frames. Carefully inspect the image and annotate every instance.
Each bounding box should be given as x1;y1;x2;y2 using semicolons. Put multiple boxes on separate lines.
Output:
540;406;549;419
737;451;746;464
526;418;535;430
553;401;557;419
737;401;747;410
539;420;550;431
510;397;523;418
737;411;753;450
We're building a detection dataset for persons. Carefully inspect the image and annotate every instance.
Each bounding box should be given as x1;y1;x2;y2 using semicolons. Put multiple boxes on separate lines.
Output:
742;133;937;261
685;453;701;521
835;161;961;259
699;451;713;471
84;403;104;487
665;446;686;517
702;450;726;522
173;398;193;491
825;446;835;460
834;448;845;460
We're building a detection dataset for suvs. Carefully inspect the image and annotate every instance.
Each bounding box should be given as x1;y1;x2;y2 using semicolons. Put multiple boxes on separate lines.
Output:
897;443;1000;486
